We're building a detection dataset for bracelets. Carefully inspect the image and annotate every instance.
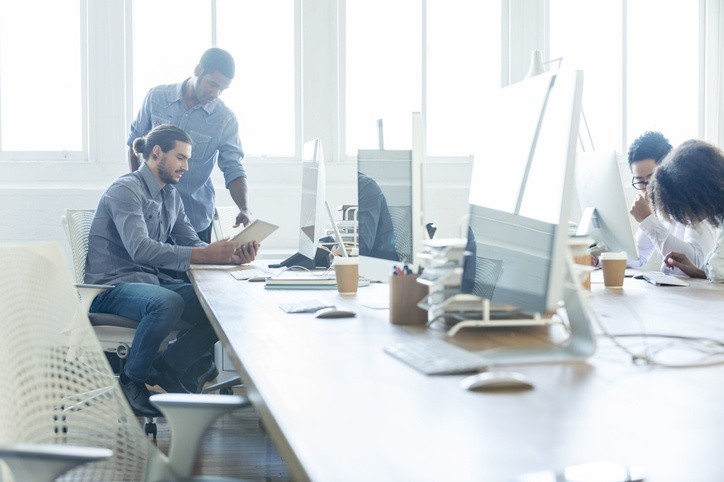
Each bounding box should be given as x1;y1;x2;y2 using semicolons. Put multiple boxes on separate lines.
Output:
239;207;251;216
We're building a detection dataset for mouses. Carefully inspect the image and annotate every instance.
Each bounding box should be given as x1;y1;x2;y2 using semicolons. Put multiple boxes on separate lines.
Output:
633;274;643;279
463;373;534;393
316;307;355;319
248;276;272;282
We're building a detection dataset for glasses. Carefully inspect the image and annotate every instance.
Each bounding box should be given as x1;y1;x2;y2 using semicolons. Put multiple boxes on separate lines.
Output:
631;175;648;191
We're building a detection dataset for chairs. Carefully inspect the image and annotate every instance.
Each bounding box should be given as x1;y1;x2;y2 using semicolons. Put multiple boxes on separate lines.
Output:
64;202;179;439
0;237;258;482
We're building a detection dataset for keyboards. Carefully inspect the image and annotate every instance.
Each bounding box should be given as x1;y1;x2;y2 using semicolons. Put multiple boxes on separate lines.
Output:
643;274;689;287
384;339;491;375
280;298;337;313
231;270;266;280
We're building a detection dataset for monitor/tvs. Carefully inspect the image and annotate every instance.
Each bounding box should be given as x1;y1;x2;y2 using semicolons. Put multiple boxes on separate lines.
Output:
297;71;639;366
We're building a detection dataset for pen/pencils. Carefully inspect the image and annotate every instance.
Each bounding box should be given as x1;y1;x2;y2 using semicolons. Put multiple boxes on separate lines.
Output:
393;263;423;275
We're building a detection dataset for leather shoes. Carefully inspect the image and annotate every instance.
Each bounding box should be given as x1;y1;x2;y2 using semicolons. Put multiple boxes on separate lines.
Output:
145;360;193;394
118;373;161;417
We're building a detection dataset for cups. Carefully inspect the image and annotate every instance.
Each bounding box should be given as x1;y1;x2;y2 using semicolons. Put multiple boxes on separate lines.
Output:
334;256;359;295
391;274;429;325
600;252;627;288
569;236;592;293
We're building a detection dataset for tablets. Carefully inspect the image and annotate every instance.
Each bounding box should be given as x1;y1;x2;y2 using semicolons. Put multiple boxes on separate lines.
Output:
229;220;279;248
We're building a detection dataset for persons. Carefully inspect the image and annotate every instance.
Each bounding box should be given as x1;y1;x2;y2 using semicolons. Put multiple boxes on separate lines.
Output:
645;139;724;282
591;130;703;276
84;124;260;419
358;170;401;260
124;48;253;281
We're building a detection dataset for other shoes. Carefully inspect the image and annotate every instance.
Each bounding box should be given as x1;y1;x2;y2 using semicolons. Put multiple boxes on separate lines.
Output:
182;362;220;394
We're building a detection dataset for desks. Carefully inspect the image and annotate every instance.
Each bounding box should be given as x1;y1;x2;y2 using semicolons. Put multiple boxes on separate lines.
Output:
187;242;724;482
